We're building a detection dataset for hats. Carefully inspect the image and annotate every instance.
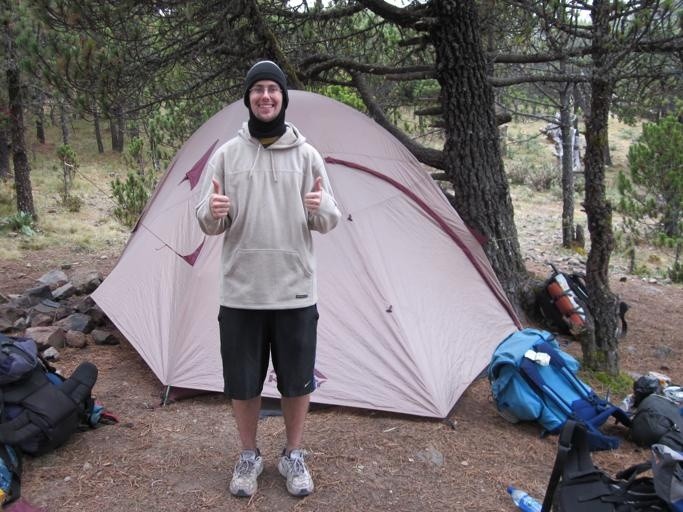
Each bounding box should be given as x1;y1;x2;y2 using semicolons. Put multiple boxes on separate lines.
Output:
244;60;288;109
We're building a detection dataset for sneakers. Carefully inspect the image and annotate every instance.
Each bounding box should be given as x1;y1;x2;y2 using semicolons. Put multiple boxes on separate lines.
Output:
229;448;263;496
277;447;314;497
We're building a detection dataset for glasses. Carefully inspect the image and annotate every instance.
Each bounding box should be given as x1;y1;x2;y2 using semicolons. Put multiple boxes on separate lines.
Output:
249;86;283;94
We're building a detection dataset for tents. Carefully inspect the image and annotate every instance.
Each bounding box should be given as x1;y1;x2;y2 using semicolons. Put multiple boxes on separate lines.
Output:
90;88;522;419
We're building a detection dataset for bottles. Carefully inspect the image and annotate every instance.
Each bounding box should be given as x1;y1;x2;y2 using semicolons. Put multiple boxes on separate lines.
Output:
507;485;542;512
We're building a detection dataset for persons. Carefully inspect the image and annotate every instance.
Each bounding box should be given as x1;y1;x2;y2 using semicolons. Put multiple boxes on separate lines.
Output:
195;60;341;497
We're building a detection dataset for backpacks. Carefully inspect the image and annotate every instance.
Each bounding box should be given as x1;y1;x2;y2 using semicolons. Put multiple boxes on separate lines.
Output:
0;333;98;506
538;272;589;341
488;328;633;451
541;394;683;512
